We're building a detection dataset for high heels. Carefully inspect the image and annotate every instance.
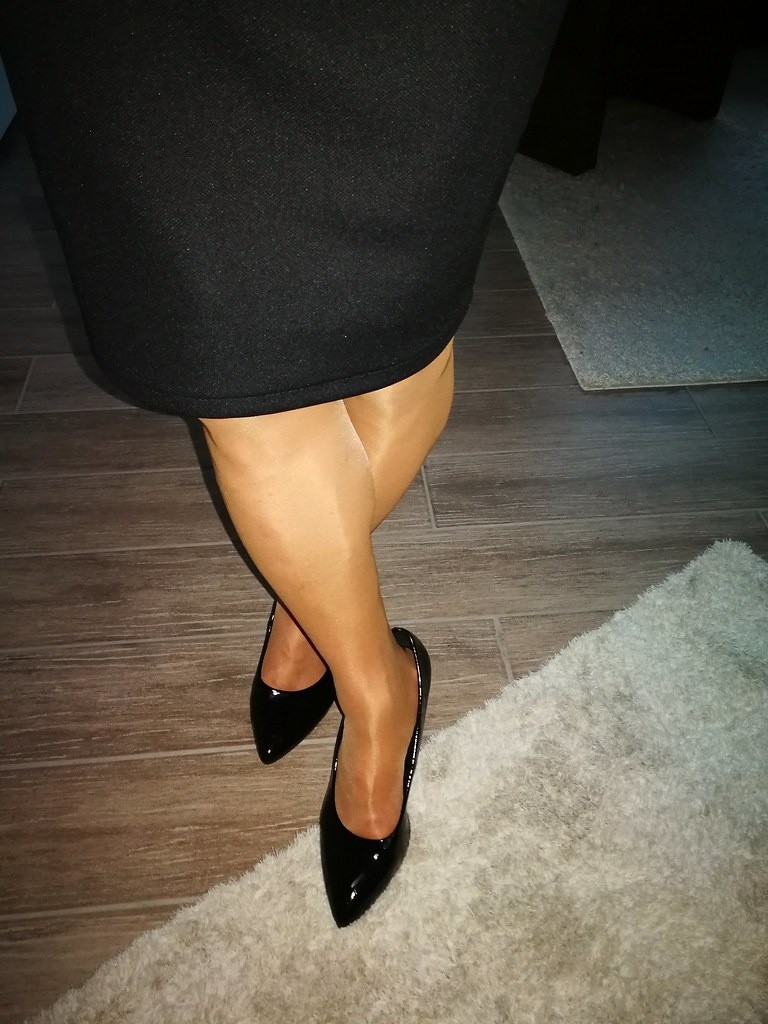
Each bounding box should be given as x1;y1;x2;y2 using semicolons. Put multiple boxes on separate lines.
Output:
319;624;435;931
246;595;336;766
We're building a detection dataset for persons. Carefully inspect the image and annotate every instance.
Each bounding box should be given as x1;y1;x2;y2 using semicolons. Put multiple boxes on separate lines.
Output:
1;0;569;930
